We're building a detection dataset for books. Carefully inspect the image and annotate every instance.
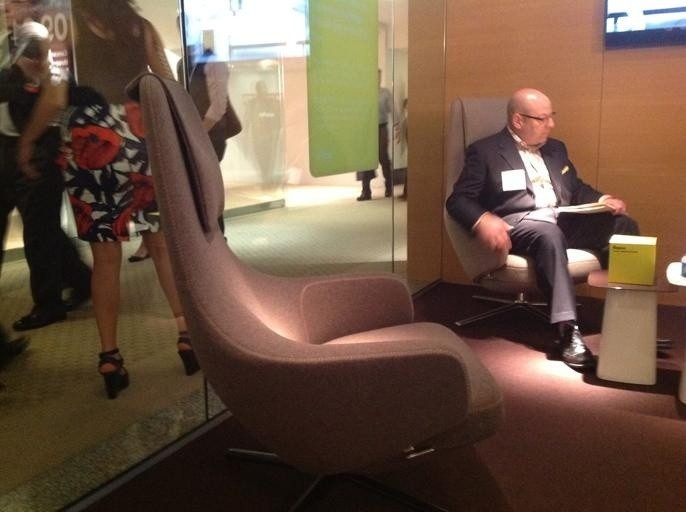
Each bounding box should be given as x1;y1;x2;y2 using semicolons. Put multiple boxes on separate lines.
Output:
556;202;630;218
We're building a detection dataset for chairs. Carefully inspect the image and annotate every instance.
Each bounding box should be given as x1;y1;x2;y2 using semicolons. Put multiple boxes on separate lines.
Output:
125;73;504;512
444;97;602;329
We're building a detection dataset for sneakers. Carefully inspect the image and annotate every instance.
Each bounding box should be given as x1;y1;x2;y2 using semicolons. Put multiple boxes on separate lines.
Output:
129;252;150;261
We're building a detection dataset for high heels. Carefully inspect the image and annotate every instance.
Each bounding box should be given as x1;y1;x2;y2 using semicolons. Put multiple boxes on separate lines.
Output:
177;337;202;376
98;348;130;400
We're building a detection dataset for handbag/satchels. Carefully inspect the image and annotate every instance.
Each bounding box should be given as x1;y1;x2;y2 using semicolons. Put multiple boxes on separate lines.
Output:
224;98;242;140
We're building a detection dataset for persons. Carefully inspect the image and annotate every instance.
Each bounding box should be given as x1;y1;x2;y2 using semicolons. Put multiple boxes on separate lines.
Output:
398;98;408;201
446;87;675;370
242;80;282;195
1;1;242;401
355;67;403;202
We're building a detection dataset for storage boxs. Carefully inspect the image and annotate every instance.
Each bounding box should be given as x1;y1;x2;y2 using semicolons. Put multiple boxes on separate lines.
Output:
608;234;658;286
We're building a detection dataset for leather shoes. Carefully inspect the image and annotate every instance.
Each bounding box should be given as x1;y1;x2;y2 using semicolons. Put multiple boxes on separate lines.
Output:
554;325;595;368
386;187;392;197
65;275;92;312
11;299;69;330
0;335;31;365
358;190;373;200
655;337;675;350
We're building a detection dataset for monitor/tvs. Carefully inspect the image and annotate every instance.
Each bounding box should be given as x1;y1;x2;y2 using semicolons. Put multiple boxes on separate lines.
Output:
604;0;686;49
181;0;310;63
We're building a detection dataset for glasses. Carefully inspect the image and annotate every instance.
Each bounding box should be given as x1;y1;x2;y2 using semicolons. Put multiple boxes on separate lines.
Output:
518;111;556;122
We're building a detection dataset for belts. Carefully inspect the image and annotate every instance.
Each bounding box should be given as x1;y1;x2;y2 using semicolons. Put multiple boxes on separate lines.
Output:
379;122;388;127
